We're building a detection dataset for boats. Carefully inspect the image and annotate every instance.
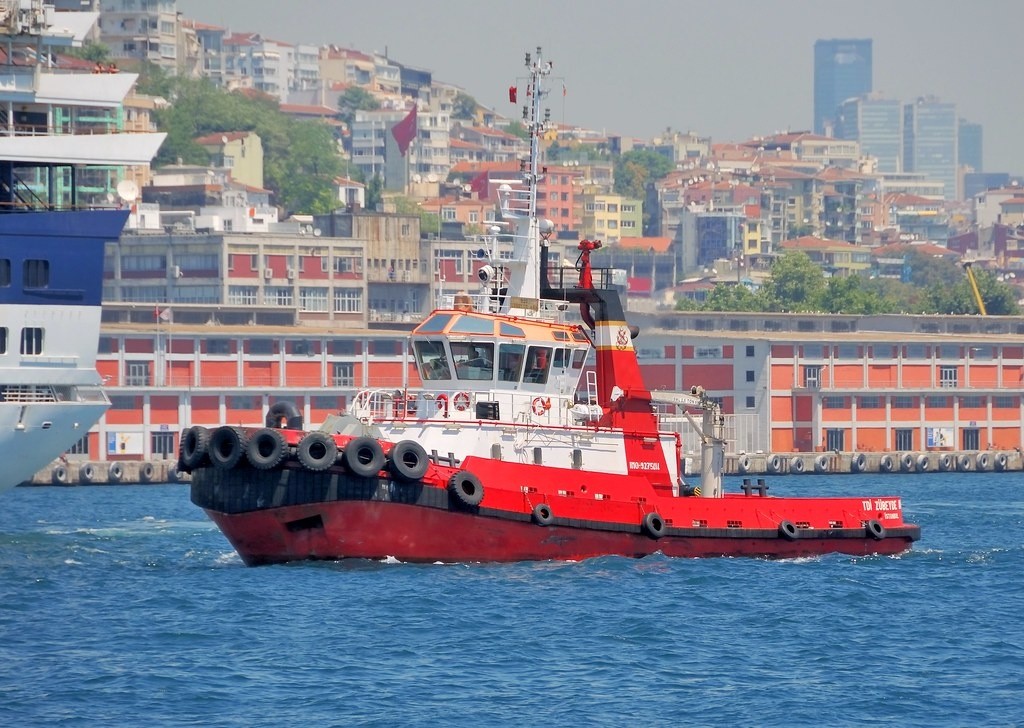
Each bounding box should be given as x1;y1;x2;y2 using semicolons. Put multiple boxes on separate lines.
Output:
175;43;920;569
0;3;170;495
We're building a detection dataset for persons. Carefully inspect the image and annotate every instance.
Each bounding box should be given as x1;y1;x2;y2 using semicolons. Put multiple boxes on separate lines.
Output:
107;62;120;74
115;201;123;210
92;60;105;74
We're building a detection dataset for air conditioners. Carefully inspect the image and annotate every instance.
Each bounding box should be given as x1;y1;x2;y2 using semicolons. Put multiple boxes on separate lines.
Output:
263;268;273;279
286;269;295;279
171;265;179;279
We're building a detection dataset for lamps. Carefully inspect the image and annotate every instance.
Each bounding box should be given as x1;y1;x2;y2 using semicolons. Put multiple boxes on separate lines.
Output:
476;265;495;283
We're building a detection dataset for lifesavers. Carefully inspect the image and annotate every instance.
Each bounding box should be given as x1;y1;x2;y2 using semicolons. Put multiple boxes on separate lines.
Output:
140;462;154;480
246;427;289;470
642;512;666;540
738;452;1007;473
178;425;209;471
296;429;338;473
533;503;554;526
52;465;68;483
454;393;470;411
80;462;94;480
448;469;485;509
108;461;123;480
779;519;799;541
533;398;546;415
388;440;429;481
866;519;886;540
208;425;246;471
343;436;385;478
437;394;448;410
265;400;303;430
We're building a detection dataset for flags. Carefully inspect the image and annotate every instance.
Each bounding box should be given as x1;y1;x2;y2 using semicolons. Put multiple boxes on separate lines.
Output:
470;170;488;200
509;86;518;104
526;82;532;97
391;104;417;157
